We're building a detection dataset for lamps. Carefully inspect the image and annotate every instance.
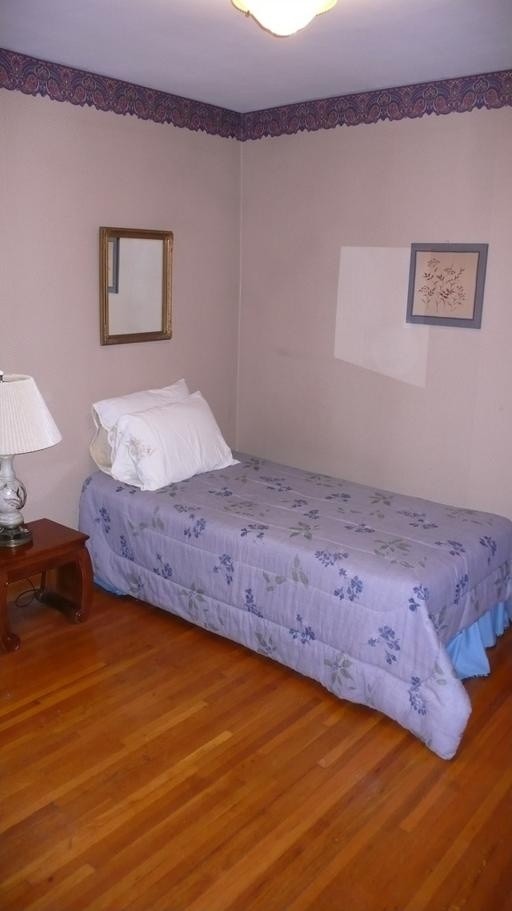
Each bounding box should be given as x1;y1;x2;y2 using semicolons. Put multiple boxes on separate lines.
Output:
0;370;62;548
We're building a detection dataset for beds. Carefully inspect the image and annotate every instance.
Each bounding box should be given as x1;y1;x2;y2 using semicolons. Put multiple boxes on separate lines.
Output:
79;449;512;761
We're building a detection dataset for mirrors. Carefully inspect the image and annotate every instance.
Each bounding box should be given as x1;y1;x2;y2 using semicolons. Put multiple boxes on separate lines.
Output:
98;226;174;346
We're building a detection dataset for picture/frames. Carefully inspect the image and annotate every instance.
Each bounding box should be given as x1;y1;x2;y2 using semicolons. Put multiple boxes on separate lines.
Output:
405;242;489;329
107;236;120;294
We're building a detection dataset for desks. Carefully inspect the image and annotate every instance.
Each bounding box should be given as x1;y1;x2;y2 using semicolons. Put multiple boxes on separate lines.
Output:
0;518;94;654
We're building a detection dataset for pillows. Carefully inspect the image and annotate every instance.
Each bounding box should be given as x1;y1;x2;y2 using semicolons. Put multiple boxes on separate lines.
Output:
89;378;241;491
